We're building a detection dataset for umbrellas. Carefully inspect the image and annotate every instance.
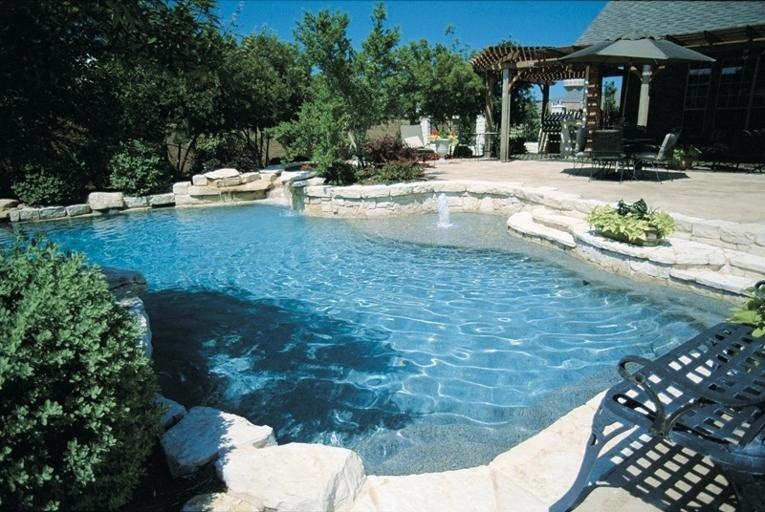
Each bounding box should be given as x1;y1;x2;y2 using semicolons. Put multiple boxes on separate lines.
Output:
558;25;718;124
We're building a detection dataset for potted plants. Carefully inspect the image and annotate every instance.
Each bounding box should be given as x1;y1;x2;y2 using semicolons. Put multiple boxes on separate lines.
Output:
672;143;703;170
586;197;676;246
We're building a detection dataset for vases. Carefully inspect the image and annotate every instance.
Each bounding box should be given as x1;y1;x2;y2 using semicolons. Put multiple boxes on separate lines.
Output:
436;139;449;160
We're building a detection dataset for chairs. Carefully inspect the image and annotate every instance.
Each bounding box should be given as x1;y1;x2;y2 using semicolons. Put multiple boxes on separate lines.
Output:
549;323;765;512
404;136;435;168
560;122;677;184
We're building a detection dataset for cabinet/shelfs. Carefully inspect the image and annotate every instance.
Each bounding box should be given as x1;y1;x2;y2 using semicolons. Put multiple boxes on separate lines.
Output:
681;54;765;129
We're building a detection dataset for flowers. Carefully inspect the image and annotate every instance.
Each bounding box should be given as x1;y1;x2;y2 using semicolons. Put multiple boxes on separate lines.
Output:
430;125;459;146
